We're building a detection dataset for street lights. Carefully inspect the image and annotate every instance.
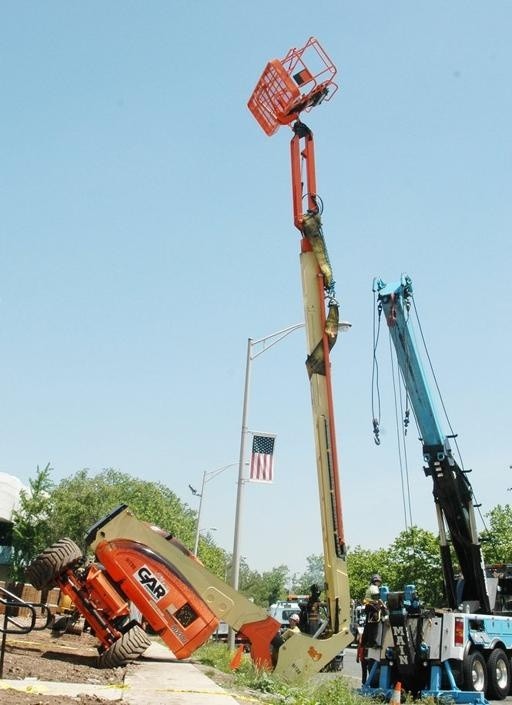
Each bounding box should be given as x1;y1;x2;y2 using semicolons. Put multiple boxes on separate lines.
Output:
230;320;353;593
193;461;250;558
199;526;219;533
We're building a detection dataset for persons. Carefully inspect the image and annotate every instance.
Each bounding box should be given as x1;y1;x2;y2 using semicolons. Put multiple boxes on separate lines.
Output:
296;584;323;634
273;612;302;667
364;574;388;648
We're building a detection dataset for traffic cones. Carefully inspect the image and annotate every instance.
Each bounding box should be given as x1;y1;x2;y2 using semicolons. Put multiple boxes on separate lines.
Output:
388;681;402;705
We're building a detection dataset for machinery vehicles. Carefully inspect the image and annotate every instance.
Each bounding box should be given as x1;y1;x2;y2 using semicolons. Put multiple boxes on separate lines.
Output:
360;270;512;705
23;32;359;688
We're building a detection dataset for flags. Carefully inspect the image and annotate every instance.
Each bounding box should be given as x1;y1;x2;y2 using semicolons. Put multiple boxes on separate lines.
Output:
247;432;277;481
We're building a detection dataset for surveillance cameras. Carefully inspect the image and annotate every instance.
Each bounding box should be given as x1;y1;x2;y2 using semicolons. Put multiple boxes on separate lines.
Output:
188;484;197;493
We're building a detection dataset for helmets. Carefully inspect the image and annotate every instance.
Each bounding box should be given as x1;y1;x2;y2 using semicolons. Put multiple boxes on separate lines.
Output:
290;614;300;623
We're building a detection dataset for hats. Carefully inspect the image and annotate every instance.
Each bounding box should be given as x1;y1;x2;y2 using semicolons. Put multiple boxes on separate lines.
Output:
372;576;381;582
